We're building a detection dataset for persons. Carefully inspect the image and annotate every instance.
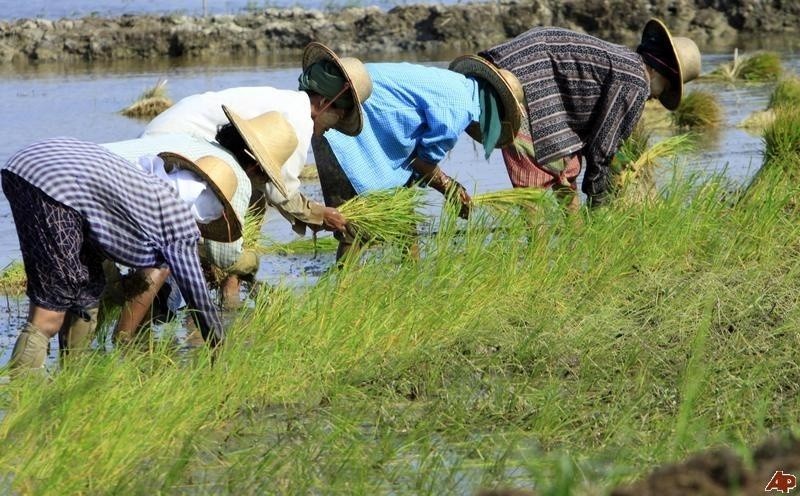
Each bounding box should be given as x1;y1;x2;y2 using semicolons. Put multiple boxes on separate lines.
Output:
477;16;701;256
138;39;373;314
89;104;299;354
298;53;524;275
0;134;244;394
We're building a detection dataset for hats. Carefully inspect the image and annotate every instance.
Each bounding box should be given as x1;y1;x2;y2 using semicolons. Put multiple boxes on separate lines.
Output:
221;103;299;202
642;19;702;112
301;41;372;139
156;151;244;244
448;53;524;150
